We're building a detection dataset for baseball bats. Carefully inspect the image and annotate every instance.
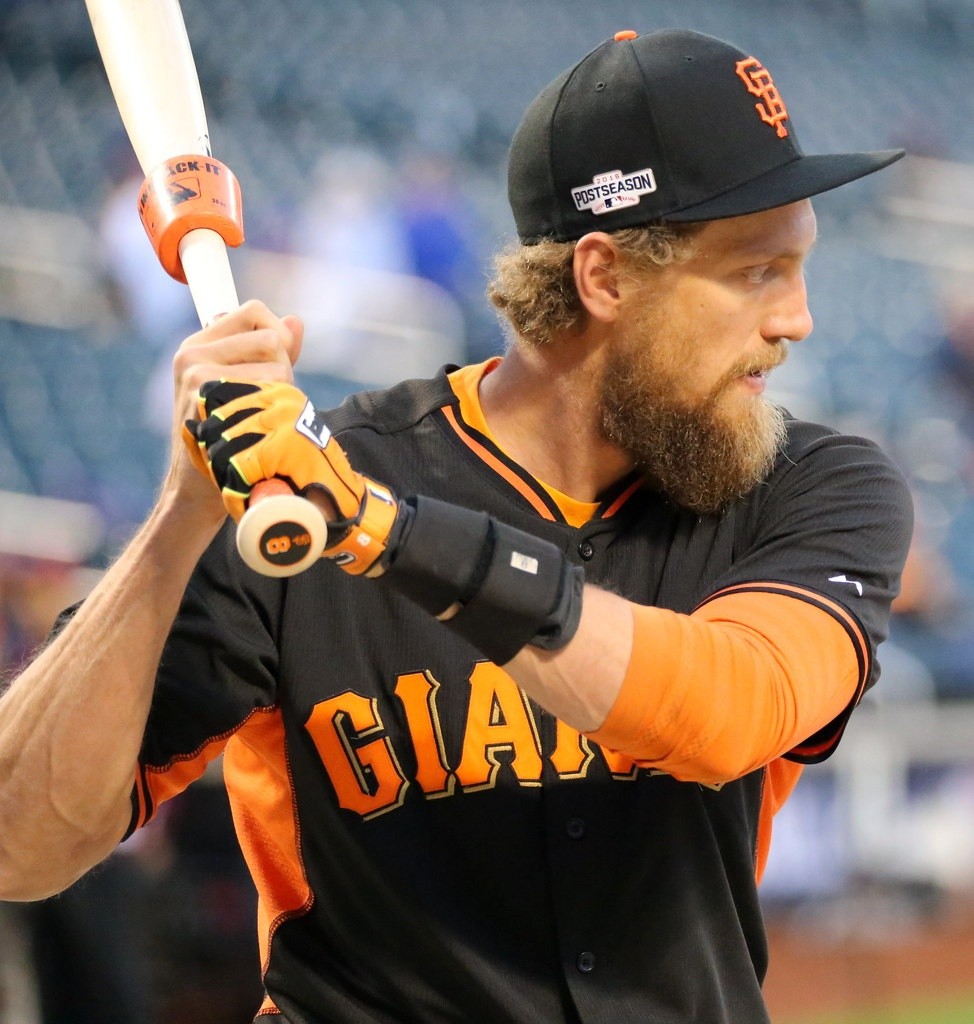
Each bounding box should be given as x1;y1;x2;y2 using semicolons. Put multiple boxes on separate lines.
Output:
84;0;331;577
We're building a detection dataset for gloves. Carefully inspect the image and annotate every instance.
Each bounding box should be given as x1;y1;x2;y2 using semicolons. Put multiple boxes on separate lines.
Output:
184;373;402;578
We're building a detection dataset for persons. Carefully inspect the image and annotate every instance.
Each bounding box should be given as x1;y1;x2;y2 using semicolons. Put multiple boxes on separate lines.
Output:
0;32;914;1023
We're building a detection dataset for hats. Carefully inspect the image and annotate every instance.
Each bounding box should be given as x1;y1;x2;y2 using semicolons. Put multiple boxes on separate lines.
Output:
507;31;905;247
